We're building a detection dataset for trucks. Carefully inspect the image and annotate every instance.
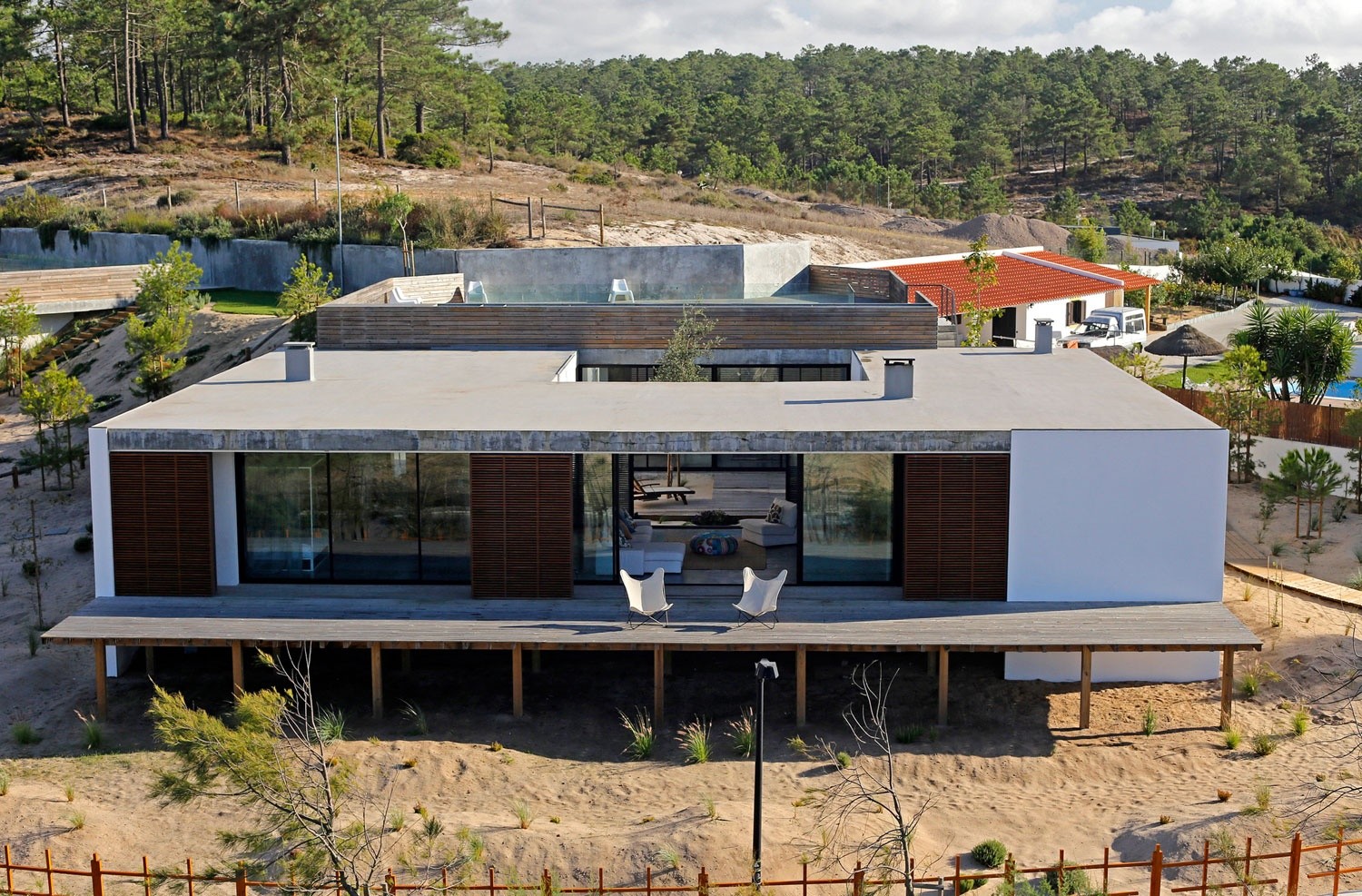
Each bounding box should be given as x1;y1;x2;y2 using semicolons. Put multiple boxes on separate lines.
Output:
1056;306;1147;357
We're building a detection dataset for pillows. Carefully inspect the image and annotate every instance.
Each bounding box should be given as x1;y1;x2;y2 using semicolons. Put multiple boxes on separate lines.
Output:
598;507;637;547
765;502;783;524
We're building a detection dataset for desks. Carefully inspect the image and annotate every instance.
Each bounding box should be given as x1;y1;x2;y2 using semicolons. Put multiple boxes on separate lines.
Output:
689;530;739;555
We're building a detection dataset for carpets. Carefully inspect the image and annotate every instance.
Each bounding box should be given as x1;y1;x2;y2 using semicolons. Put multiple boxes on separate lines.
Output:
668;535;767;570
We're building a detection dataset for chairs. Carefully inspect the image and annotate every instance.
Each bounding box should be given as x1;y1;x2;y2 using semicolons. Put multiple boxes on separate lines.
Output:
733;566;788;629
633;477;695;505
621;567;673;629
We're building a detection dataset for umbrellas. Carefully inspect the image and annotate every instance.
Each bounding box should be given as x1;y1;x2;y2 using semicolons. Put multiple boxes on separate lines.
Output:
1144;324;1229;389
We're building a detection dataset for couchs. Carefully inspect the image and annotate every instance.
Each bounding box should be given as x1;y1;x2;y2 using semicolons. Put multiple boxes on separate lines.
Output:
739;498;798;546
596;519;685;575
